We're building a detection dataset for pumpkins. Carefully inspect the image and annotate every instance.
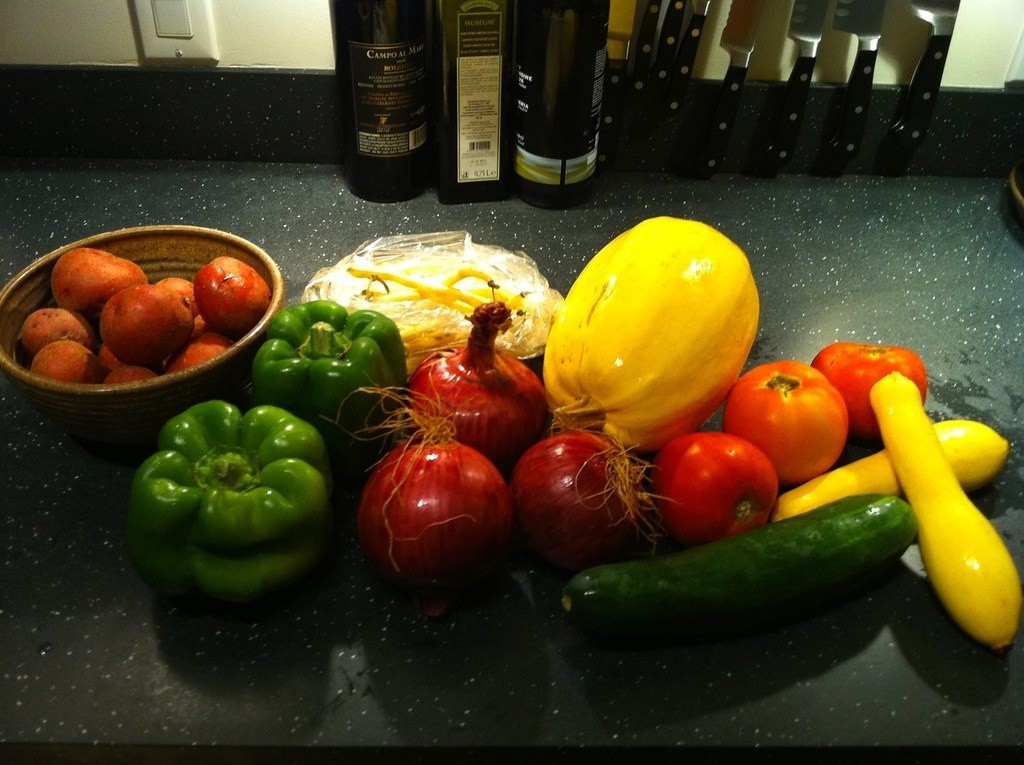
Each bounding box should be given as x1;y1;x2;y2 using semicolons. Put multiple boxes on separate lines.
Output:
542;215;761;458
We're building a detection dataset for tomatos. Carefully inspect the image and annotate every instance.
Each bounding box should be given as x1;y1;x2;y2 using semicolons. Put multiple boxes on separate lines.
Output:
649;343;928;546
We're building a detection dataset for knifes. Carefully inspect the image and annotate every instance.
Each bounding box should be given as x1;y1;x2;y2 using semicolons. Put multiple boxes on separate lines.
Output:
758;0;831;173
623;0;711;177
872;0;963;177
694;1;768;176
814;0;889;176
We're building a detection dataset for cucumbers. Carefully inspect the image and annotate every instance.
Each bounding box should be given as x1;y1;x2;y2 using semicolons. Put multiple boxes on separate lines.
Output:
563;493;918;642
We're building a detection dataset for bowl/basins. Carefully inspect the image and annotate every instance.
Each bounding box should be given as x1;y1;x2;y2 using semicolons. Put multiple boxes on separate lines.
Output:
0;218;284;454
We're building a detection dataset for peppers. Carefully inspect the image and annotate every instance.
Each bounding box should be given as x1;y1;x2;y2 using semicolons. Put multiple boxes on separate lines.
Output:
126;297;406;601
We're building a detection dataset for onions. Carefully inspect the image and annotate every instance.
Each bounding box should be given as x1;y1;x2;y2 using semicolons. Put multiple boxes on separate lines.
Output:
359;301;637;591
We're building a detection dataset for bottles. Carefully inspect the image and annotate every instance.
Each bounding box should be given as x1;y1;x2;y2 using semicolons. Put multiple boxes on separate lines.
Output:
332;0;439;202
430;0;516;203
503;0;611;209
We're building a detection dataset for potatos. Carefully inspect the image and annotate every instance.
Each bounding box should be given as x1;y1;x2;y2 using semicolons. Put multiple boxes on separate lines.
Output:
21;247;271;386
